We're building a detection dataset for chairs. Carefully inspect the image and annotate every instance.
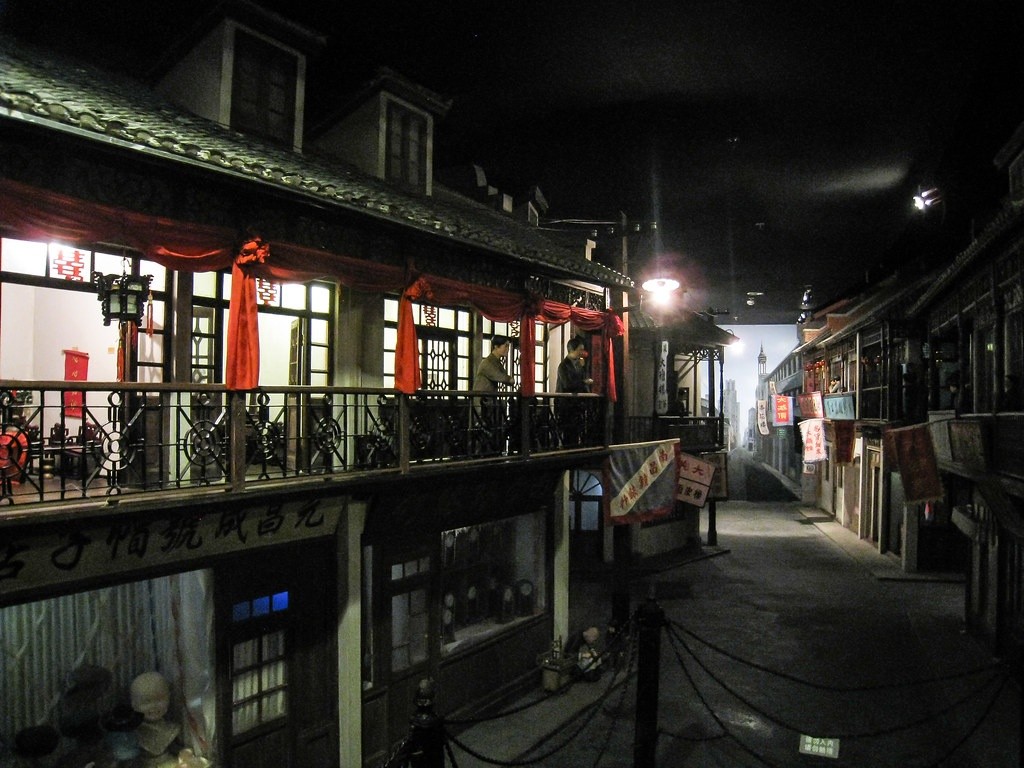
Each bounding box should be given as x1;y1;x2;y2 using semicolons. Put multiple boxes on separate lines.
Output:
26;422;99;478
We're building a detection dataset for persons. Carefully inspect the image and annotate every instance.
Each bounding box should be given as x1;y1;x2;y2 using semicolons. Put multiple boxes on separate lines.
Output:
903;362;918;413
130;672;180;754
829;375;841;393
554;338;594;444
472;334;515;454
